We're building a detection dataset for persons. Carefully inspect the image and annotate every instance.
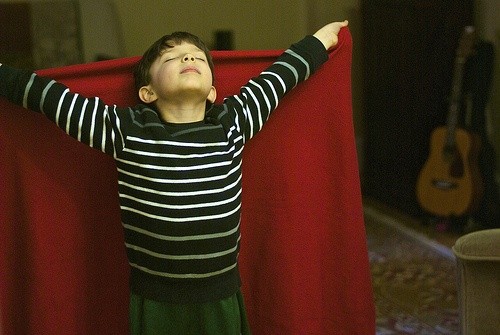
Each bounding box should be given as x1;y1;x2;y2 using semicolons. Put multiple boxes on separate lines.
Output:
0;19;349;335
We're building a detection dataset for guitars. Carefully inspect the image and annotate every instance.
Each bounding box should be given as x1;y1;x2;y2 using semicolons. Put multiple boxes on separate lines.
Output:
416;27;483;217
